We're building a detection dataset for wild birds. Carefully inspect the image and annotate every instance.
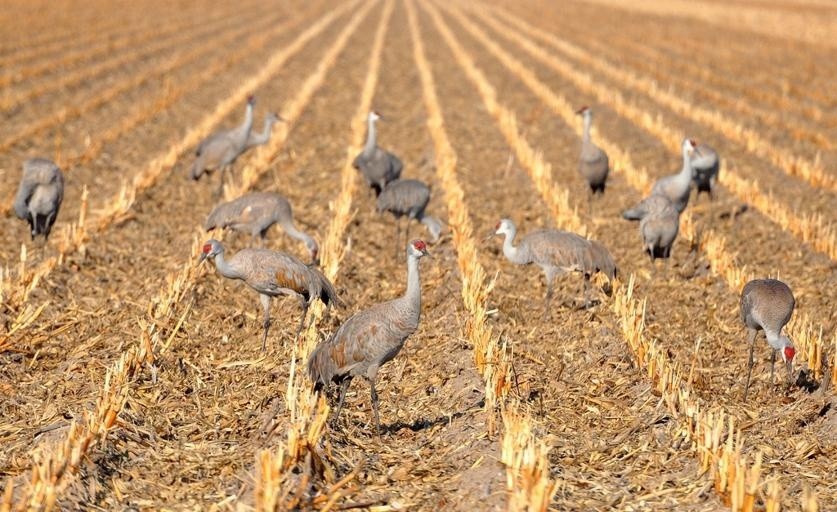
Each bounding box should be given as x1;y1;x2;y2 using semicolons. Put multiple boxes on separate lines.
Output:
194;240;337;353
192;95;285;204
205;192;318;260
351;110;404;199
640;195;679;264
691;145;718;202
308;239;436;438
482;218;617;322
622;138;703;221
740;279;795;403
13;159;64;246
576;106;608;196
375;179;443;264
